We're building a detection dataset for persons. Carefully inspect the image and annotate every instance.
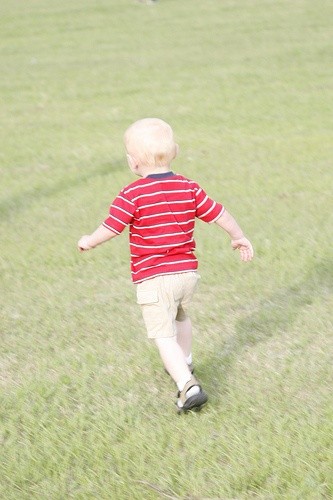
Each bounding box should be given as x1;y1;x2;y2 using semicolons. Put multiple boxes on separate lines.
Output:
79;118;256;416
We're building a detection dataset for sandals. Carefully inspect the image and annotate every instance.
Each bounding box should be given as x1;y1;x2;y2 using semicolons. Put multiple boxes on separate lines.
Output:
176;378;208;410
